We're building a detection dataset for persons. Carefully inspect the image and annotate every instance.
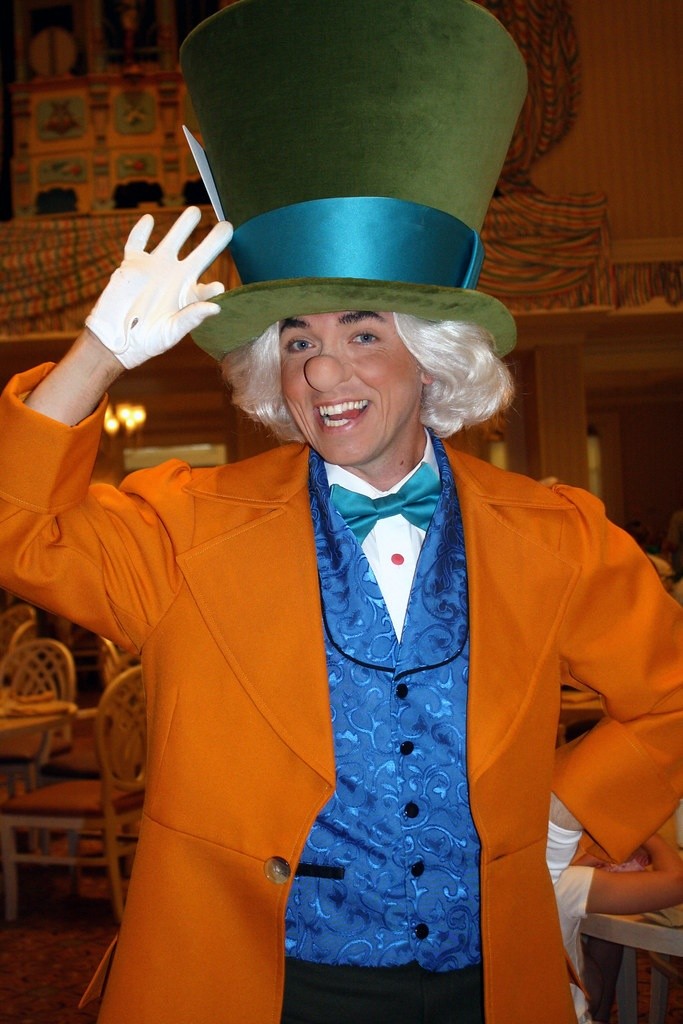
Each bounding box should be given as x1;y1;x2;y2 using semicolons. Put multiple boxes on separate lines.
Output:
552;832;683;1023
0;0;683;1024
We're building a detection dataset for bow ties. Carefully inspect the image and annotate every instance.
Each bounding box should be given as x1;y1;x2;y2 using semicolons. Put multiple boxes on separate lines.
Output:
328;462;442;547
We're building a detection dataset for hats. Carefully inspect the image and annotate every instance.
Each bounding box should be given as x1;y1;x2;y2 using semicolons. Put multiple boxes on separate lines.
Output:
179;0;527;360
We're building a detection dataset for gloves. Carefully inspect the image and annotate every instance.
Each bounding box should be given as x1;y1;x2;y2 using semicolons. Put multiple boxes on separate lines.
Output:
83;205;235;370
545;820;582;885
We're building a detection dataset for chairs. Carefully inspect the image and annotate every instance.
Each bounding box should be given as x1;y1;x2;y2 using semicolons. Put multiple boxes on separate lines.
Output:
0;603;148;923
